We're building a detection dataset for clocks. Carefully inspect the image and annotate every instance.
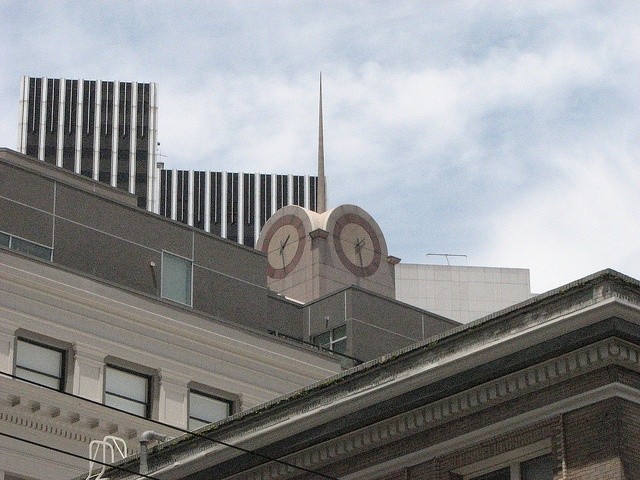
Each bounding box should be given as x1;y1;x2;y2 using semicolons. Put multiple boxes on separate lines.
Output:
332;212;382;278
261;214;307;280
